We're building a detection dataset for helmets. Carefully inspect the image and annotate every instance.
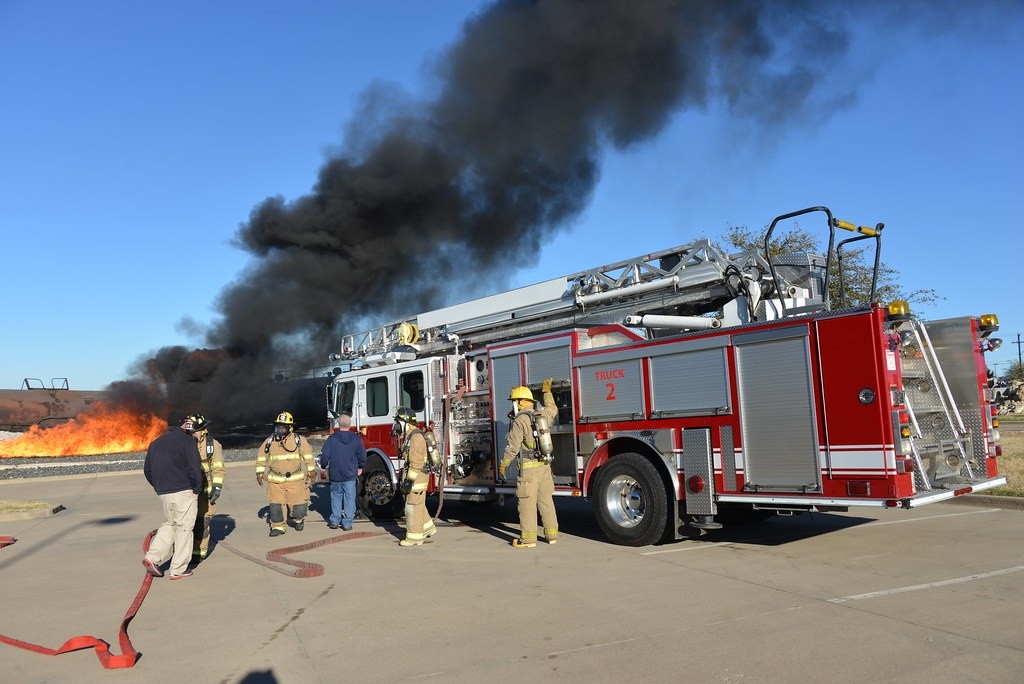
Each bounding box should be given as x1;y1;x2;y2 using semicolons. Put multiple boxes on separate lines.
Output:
393;407;419;428
184;412;212;432
506;386;538;403
274;413;296;425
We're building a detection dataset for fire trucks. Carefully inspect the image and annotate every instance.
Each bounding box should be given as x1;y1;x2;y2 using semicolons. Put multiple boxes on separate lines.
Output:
267;203;1008;548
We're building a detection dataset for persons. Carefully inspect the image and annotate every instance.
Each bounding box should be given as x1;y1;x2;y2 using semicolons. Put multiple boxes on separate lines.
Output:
321;414;367;532
142;408;226;581
499;377;561;548
389;406;437;547
256;411;317;537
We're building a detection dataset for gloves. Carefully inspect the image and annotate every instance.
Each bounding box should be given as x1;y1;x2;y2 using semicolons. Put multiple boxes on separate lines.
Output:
207;483;222;504
256;472;266;486
499;461;507;480
400;479;414;495
305;469;317;486
542;378;554;392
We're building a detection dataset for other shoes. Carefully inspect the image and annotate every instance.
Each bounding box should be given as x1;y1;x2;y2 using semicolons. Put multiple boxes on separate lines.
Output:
189;554;205;567
142;558;163;576
400;537;425;546
513;538;534;547
424;524;436;538
269;529;284;537
343;525;352;531
294;521;304;531
329;522;338;529
169;567;194;579
548;540;558;544
